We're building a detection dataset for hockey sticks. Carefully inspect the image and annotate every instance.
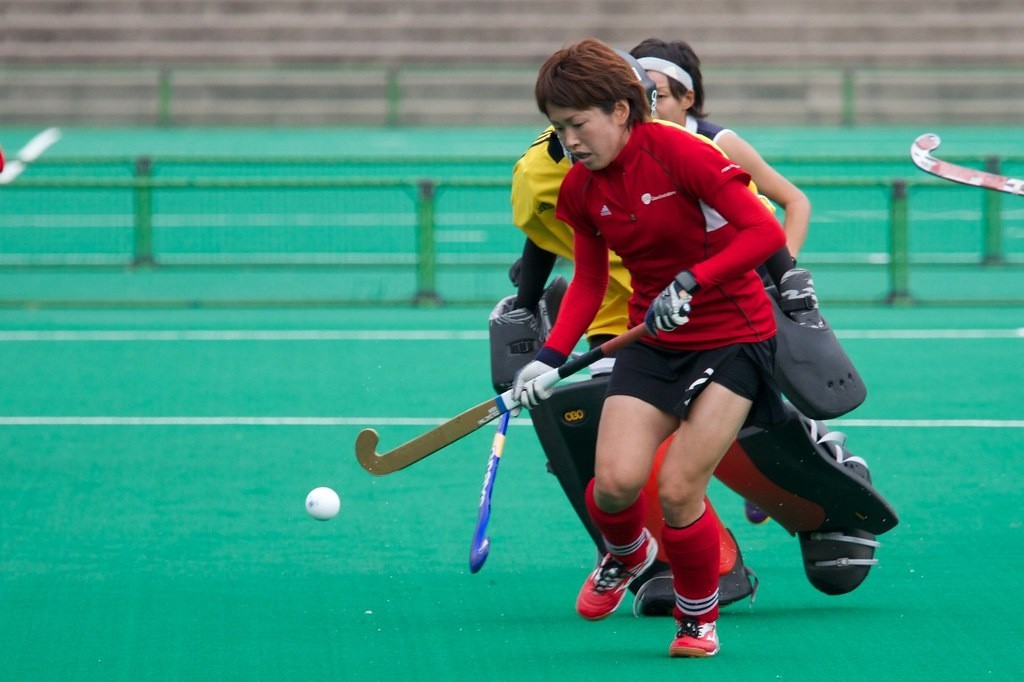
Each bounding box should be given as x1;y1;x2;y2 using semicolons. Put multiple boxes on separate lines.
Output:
468;387;514;576
355;301;688;476
909;131;1024;197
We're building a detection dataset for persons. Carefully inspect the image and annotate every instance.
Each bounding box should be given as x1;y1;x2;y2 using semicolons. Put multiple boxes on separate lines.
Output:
498;37;877;659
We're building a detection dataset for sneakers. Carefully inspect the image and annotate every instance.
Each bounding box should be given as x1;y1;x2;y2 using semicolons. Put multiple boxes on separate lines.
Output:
576;527;658;621
670;616;719;657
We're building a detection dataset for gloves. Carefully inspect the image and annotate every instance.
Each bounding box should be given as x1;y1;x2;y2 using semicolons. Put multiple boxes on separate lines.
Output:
510;359;556;418
645;281;693;337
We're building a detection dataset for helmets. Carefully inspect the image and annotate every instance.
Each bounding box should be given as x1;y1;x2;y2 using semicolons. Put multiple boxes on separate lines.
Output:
609;47;657;120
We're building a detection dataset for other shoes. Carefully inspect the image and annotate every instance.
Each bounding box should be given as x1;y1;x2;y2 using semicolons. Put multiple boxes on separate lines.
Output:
745;499;769;523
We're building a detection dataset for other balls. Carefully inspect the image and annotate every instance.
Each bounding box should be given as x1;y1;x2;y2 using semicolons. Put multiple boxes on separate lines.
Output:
302;485;342;521
